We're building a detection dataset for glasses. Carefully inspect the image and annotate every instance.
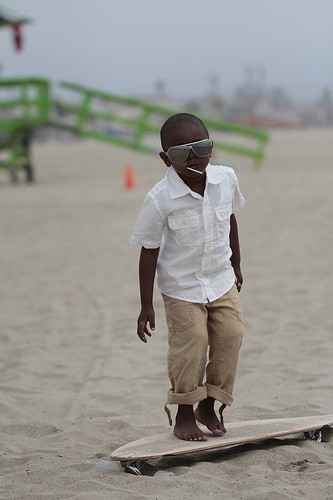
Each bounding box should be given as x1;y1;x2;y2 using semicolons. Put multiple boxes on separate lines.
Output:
164;137;214;164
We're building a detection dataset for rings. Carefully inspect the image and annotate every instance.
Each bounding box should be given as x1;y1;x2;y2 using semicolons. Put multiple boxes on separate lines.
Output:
237;283;241;285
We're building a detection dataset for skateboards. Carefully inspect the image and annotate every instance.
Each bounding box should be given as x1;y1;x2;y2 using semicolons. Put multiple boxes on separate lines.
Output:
112;412;333;465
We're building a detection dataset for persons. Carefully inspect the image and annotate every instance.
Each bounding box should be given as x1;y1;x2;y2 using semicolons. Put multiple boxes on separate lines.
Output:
128;112;247;441
